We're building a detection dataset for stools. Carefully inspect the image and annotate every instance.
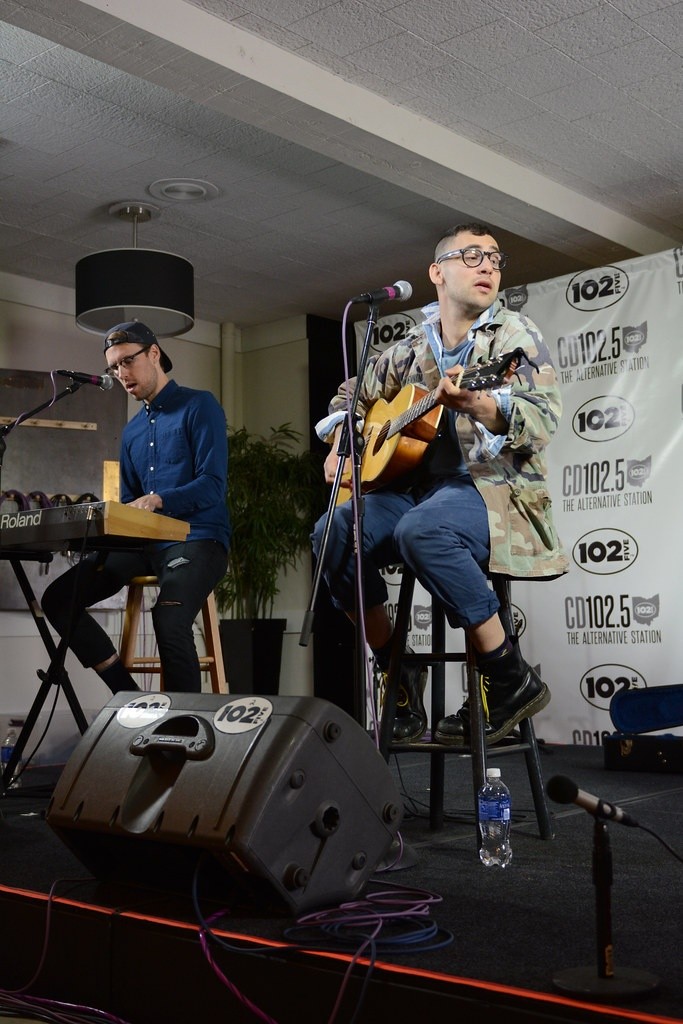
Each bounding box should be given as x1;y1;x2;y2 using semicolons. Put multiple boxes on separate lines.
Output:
118;574;227;694
379;564;555;850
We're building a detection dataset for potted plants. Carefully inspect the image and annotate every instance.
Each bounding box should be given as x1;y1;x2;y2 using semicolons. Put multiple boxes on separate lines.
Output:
214;421;320;695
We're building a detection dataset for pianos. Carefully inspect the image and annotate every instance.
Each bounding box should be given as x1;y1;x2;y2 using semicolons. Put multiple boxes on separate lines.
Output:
0;500;191;800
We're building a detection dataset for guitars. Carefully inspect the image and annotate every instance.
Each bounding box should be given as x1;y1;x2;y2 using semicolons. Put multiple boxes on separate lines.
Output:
330;346;525;508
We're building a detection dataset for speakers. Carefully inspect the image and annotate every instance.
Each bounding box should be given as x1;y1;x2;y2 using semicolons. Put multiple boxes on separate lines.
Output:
46;690;407;916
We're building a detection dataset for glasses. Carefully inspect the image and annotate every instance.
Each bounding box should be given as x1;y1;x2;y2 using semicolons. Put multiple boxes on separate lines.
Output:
436;249;509;270
105;343;152;378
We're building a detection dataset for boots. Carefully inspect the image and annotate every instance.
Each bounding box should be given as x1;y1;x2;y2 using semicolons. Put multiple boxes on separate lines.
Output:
434;636;551;746
378;642;428;743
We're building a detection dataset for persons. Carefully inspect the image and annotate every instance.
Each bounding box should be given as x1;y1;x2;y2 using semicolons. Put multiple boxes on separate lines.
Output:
310;223;570;745
41;322;232;696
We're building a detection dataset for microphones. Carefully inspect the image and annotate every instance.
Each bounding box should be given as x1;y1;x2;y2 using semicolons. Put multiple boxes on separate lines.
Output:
351;280;412;304
545;775;637;825
57;369;113;391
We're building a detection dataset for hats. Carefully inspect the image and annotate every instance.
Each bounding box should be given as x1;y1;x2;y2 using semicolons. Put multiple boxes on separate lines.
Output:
104;322;173;374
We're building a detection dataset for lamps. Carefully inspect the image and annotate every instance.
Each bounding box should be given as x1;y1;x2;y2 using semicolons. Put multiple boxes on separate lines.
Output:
74;202;195;339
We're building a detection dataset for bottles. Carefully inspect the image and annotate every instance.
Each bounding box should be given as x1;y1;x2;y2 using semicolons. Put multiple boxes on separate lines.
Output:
0;728;21;789
477;768;512;867
